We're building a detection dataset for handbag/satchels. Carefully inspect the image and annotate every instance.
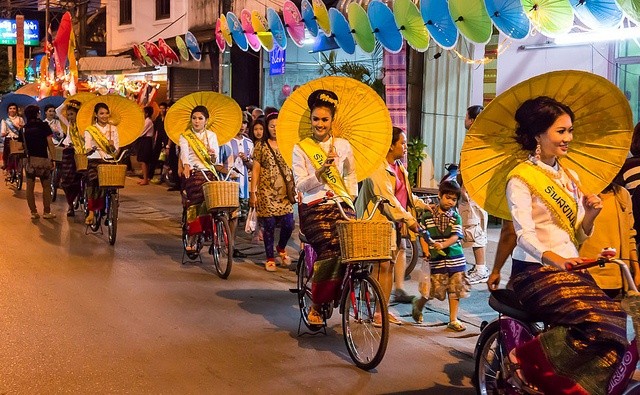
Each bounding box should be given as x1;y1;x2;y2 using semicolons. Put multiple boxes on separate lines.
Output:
286;181;296;204
157;148;166;162
25;157;53;178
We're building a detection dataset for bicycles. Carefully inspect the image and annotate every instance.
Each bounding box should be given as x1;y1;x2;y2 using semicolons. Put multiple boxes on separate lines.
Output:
81;147;132;246
471;248;639;394
291;195;396;372
46;135;66;201
2;130;26;190
398;228;421;277
179;152;253;280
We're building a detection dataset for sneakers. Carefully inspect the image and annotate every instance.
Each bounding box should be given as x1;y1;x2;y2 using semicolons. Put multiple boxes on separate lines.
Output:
67;207;74;216
73;201;79;209
43;213;56;219
31;213;40;219
465;265;490;285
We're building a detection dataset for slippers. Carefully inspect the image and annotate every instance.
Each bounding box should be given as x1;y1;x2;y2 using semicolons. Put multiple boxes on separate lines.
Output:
185;242;196;253
372;312;382;327
412;297;423;324
227;249;246;258
85;216;94;225
308;305;324;325
503;354;545;395
447;321;466;332
276;250;291;266
266;259;276;272
388;312;402;325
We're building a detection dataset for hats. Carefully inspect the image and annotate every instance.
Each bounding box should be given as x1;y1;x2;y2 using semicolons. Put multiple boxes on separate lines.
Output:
243;111;248;123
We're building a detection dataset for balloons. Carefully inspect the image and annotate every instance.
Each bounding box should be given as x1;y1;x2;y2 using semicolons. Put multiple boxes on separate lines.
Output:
282;84;291;97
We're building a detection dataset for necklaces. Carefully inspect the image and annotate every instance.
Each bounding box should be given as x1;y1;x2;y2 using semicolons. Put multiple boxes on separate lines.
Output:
528;162;563;179
312;133;334;156
96;123;110;135
192;129;206;142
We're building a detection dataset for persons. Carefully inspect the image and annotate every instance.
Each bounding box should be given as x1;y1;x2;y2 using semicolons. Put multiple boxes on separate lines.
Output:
0;103;25;177
578;183;640;303
456;105;490;285
209;104;254;259
164;101;178;191
17;106;56;219
55;99;85;216
179;106;219;251
293;89;359;326
242;104;268;140
248;107;303;272
355;126;436;328
486;220;544;389
84;102;119;225
613;121;640;198
506;95;628;395
137;106;154;185
412;180;472;331
148;102;168;184
42;104;64;150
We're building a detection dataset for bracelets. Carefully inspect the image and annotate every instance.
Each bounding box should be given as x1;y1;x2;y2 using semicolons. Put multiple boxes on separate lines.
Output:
51;159;56;162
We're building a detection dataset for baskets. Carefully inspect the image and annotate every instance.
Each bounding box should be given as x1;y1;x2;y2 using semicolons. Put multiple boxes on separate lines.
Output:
620;289;640;361
335;220;393;264
9;140;24;154
98;164;127;189
202;181;240;213
74;154;87;171
47;147;64;161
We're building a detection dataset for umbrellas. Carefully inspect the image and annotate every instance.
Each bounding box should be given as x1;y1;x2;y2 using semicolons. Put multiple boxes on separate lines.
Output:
420;0;459;50
218;13;232;48
266;8;287;50
76;95;145;148
448;0;493;45
367;0;403;54
185;31;201;61
460;70;634;224
226;12;248;52
158;38;172;65
347;2;376;54
139;45;152;65
161;44;179;64
283;0;305;48
61;92;98;136
328;8;356;55
133;44;146;65
38;96;67;121
312;0;331;38
569;0;625;32
145;41;160;66
149;42;165;66
251;10;274;52
520;0;574;38
215;18;226;54
14;81;52;96
393;0;430;53
164;91;243;148
301;0;319;38
0;94;43;124
615;0;640;27
176;35;189;61
51;11;72;75
275;76;393;183
483;0;531;41
240;9;262;52
